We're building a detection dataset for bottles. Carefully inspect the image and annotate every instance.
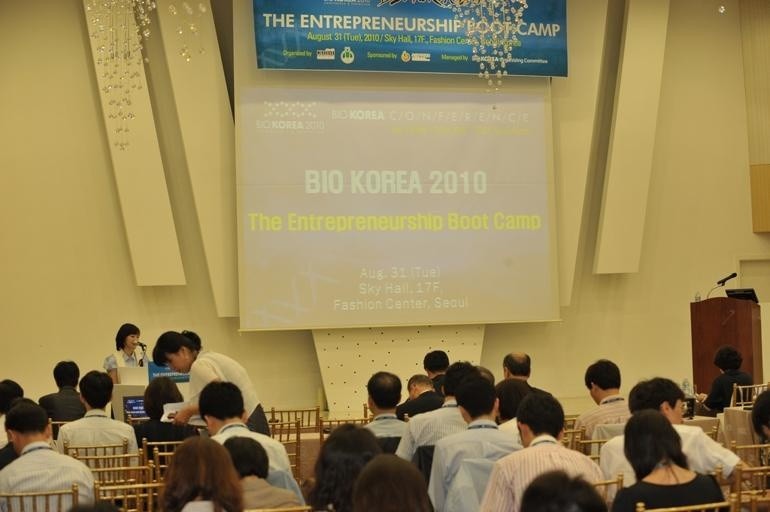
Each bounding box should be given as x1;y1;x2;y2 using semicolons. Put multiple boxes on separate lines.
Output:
682;377;690;394
695;291;699;302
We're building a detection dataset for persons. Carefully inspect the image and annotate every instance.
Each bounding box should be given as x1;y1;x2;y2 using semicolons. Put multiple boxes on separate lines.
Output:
153;331;270;436
309;346;769;511
0;361;305;511
103;323;141;384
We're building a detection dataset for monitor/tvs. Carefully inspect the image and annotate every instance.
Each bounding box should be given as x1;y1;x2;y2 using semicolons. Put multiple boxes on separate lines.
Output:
723;287;760;304
122;396;147;417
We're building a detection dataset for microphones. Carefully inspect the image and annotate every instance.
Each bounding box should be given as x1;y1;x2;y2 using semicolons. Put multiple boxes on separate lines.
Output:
716;272;738;285
132;340;148;349
